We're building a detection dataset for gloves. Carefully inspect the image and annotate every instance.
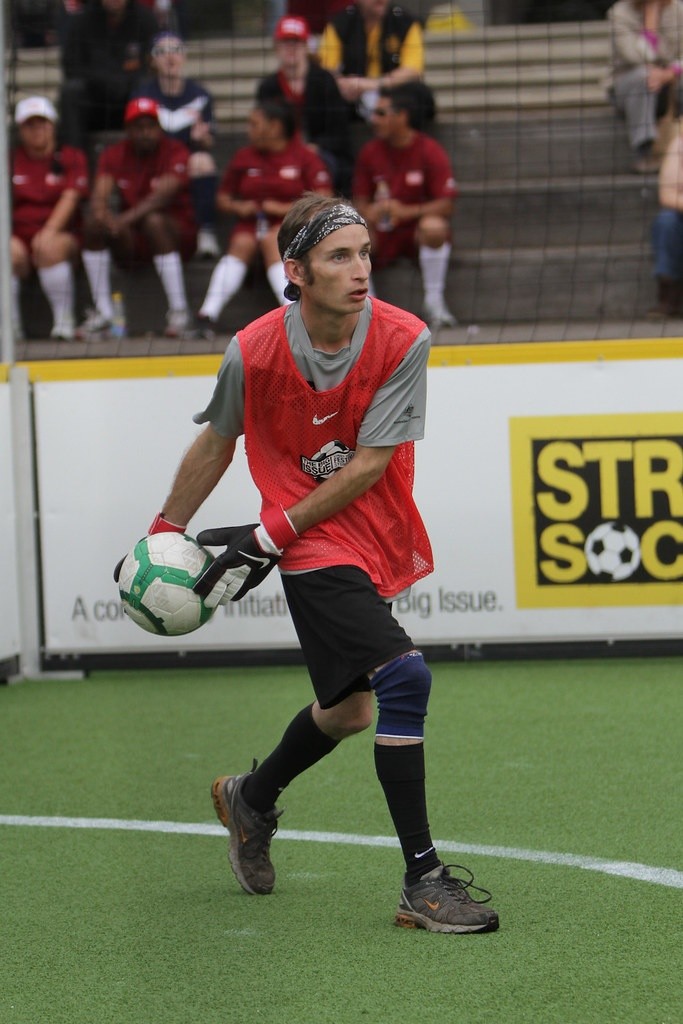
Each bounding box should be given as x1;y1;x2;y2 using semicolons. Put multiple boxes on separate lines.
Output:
192;504;299;609
114;512;187;583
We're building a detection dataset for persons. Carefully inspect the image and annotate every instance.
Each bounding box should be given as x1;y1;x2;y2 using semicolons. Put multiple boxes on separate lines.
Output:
113;195;502;935
9;0;466;344
604;0;683;317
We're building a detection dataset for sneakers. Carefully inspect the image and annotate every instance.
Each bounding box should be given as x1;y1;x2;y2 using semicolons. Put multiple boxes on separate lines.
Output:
211;758;286;895
74;308;113;344
176;315;216;342
164;307;188;337
421;302;457;330
50;314;75;342
393;861;500;934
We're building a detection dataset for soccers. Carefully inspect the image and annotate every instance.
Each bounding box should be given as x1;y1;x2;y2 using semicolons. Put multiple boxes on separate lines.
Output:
117;532;218;637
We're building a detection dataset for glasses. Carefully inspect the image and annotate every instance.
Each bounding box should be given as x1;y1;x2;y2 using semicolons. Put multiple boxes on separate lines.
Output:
369;107;385;116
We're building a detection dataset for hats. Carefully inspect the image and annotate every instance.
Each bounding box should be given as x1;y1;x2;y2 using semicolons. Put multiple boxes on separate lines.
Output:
124;97;159;124
274;16;309;41
14;96;58;125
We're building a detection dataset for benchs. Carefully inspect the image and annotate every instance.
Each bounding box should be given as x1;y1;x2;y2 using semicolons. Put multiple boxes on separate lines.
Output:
8;109;668;342
